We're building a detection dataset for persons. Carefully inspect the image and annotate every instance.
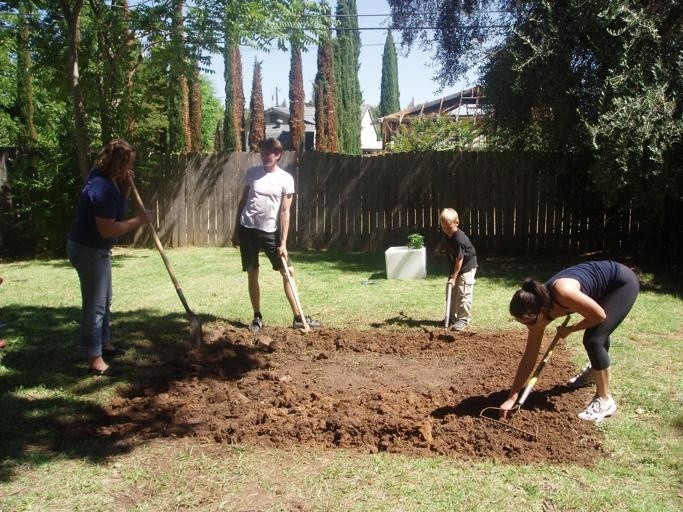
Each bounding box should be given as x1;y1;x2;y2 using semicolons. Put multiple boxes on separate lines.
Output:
500;260;640;420
66;139;155;376
231;138;321;333
441;208;478;332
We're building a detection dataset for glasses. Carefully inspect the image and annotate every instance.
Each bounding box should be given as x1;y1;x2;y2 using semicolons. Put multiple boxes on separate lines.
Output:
527;314;539;326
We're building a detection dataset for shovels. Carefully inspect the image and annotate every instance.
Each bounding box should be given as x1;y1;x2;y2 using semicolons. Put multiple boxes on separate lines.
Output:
128;175;202;350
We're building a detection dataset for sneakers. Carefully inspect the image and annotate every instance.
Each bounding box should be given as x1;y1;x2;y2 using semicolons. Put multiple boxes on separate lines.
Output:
293;319;320;329
250;317;263;331
441;318;458;325
569;362;595;387
102;348;124;356
88;365;119;376
453;319;468;330
577;395;616;421
